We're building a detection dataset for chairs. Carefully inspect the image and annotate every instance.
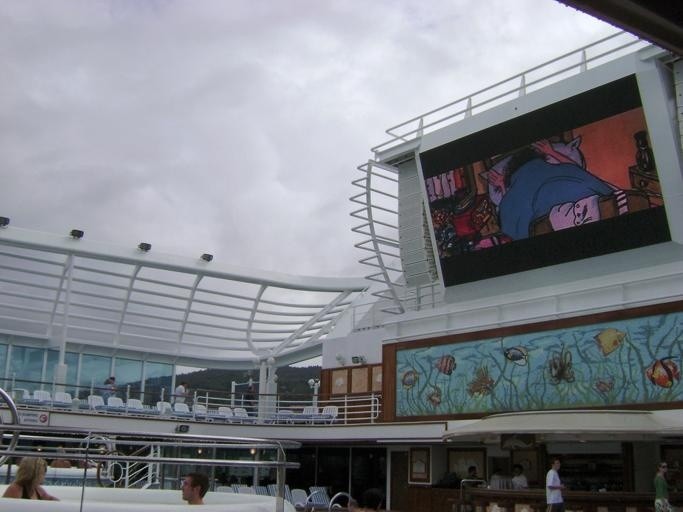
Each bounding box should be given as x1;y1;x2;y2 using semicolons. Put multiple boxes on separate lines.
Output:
11;387;80;411
81;395;250;426
215;483;344;511
276;406;338;426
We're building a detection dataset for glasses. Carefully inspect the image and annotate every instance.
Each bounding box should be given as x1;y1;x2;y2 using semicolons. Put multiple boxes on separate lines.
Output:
663;466;667;468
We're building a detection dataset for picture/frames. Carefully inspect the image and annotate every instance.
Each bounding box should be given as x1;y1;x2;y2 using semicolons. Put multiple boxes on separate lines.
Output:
510;448;542;486
445;445;490;485
406;444;433;486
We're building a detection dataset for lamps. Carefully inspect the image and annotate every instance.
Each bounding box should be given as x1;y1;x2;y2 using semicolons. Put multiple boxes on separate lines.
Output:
0;215;10;228
351;353;369;365
138;242;152;251
200;253;214;262
69;229;84;239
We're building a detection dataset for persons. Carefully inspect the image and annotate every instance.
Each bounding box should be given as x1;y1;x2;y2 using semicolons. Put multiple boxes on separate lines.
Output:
50;446;71;468
99;376;117;405
361;487;384;512
463;465;529;504
2;456;61;501
182;472;210;505
653;461;673;512
175;381;188;404
545;457;568;512
243;385;257;417
